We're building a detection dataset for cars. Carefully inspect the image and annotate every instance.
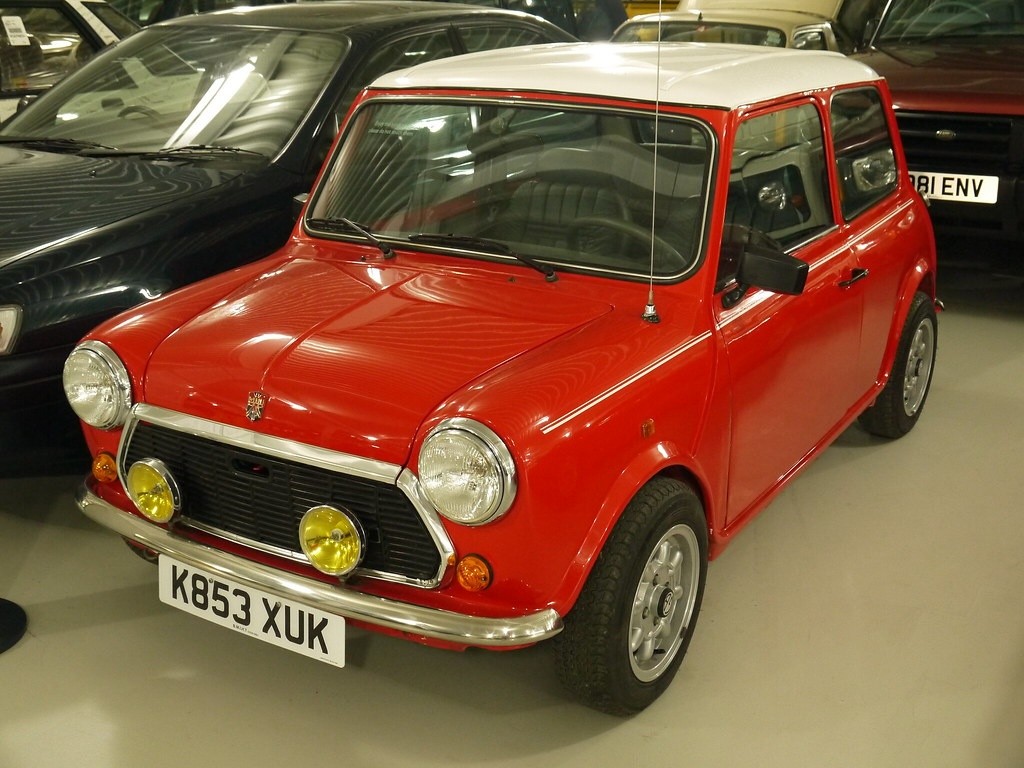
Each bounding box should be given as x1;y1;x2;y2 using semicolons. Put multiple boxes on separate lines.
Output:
60;40;939;717
3;2;1024;290
1;0;607;470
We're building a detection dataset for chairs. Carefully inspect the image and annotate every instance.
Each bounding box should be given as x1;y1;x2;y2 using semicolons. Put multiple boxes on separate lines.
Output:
496;138;635;270
0;37;67;92
651;158;803;279
